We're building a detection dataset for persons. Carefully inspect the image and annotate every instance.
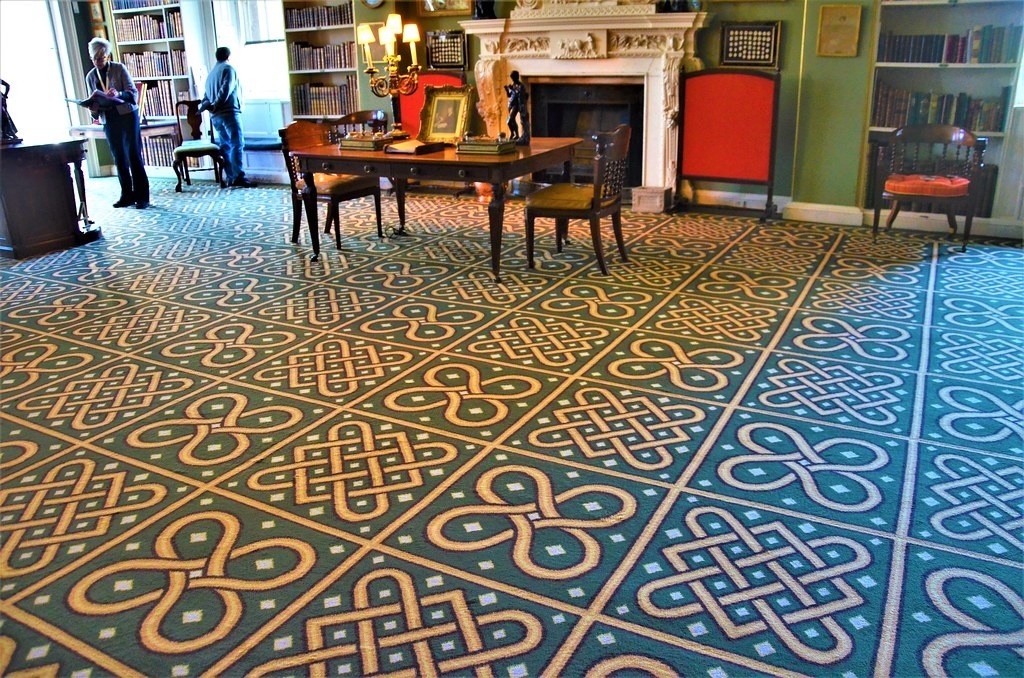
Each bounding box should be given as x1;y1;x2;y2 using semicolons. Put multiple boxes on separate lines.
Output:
504;71;525;140
200;46;254;186
85;38;150;209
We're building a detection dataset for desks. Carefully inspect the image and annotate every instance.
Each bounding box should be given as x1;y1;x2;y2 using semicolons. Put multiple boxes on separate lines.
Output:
288;137;584;283
0;134;102;261
67;115;187;179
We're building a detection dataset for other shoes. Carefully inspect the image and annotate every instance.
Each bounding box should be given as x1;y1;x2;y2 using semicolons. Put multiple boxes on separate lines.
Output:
231;177;257;188
135;200;150;209
113;196;135;208
226;181;232;187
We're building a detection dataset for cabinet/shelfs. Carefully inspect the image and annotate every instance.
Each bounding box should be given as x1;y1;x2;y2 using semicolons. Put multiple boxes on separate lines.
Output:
858;0;1024;224
280;0;396;190
109;0;205;178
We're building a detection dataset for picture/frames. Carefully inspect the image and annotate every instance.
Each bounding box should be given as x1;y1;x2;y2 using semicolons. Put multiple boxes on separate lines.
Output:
92;25;108;39
717;19;783;71
88;0;105;23
416;0;474;18
424;29;469;72
815;4;863;58
416;83;475;146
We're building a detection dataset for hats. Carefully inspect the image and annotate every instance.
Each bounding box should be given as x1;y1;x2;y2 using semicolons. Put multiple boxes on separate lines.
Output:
216;47;230;62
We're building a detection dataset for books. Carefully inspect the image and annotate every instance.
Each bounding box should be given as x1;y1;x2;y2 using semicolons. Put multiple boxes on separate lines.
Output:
65;90;125;112
289;41;355;71
111;0;199;168
286;0;353;28
292;74;357;115
876;24;1022;63
873;79;1011;132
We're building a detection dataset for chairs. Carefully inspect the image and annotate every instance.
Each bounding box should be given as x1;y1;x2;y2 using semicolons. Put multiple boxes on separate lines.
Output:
321;111;388;144
278;120;382;250
872;123;988;253
171;100;228;193
523;123;631;276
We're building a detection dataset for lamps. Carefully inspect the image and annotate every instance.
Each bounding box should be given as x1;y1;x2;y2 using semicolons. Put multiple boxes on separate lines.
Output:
357;14;430;141
